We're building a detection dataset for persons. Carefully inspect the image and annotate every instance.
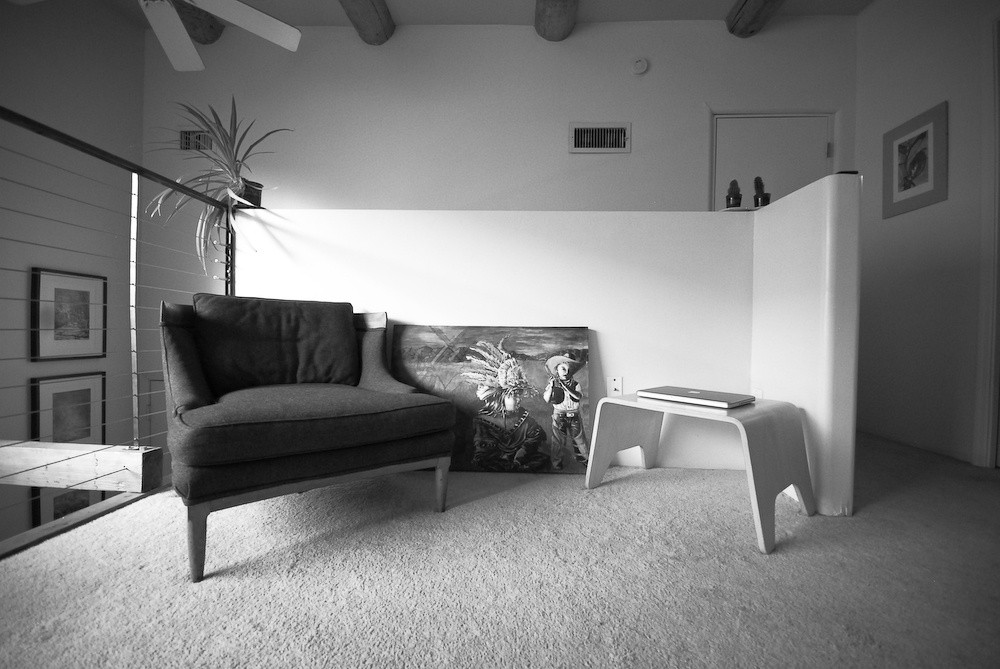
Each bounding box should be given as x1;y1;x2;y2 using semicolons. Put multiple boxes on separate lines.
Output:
460;335;590;473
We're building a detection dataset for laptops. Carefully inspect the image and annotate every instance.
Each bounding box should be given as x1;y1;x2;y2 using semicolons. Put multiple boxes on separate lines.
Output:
636;386;755;408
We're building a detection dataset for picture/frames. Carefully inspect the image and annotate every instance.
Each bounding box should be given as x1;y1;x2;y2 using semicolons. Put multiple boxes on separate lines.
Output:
30;267;108;362
31;372;106;445
31;488;105;528
881;100;948;220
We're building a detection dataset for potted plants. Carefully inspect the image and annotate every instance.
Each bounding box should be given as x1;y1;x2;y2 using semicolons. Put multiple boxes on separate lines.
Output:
726;180;742;209
754;175;770;209
144;94;296;277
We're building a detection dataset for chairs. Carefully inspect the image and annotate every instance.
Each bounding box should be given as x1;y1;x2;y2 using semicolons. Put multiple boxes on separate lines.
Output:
159;292;458;582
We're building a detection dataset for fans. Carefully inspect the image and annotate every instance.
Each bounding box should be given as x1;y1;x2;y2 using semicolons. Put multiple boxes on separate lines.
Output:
137;0;302;72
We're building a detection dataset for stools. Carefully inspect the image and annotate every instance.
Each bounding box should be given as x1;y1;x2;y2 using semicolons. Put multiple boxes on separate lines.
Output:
585;393;819;554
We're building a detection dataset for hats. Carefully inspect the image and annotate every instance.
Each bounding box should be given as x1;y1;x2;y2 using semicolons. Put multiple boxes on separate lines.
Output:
544;352;586;381
